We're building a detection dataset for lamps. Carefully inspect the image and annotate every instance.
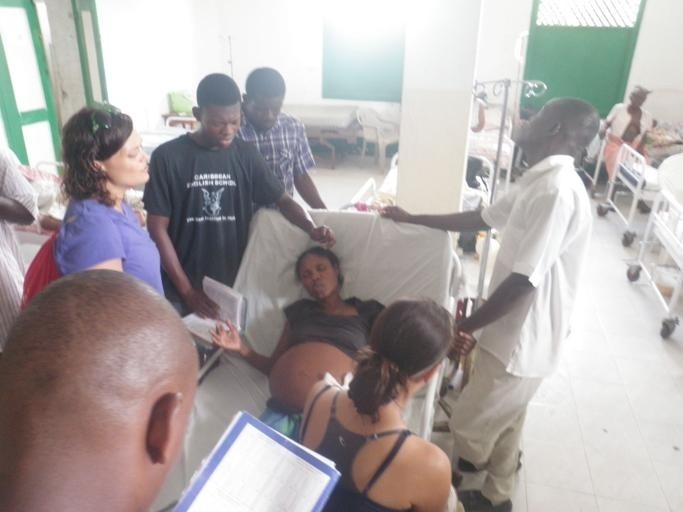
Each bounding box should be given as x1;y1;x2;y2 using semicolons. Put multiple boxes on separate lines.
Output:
354;106;400;170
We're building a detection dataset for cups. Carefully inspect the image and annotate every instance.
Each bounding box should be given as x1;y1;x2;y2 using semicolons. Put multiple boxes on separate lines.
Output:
91;103;121;135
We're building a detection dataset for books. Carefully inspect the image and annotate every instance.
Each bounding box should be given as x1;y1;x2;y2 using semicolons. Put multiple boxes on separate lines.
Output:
182;276;247;348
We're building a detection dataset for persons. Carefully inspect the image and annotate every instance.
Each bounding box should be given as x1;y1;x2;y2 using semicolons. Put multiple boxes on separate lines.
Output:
236;67;328;209
1;269;199;511
210;246;477;443
54;103;165;296
142;73;337;368
1;143;40;345
598;86;651;215
298;300;456;511
378;98;599;511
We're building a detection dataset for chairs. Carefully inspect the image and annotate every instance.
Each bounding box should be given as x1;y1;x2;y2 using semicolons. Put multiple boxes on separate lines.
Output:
458;490;512;512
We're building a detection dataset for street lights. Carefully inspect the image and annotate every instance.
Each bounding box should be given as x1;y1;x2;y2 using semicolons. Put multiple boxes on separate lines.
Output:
20;233;62;311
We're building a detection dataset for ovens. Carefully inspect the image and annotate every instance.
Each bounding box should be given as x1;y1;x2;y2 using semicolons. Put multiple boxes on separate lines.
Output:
586;119;682;341
154;204;461;511
282;103;359;169
